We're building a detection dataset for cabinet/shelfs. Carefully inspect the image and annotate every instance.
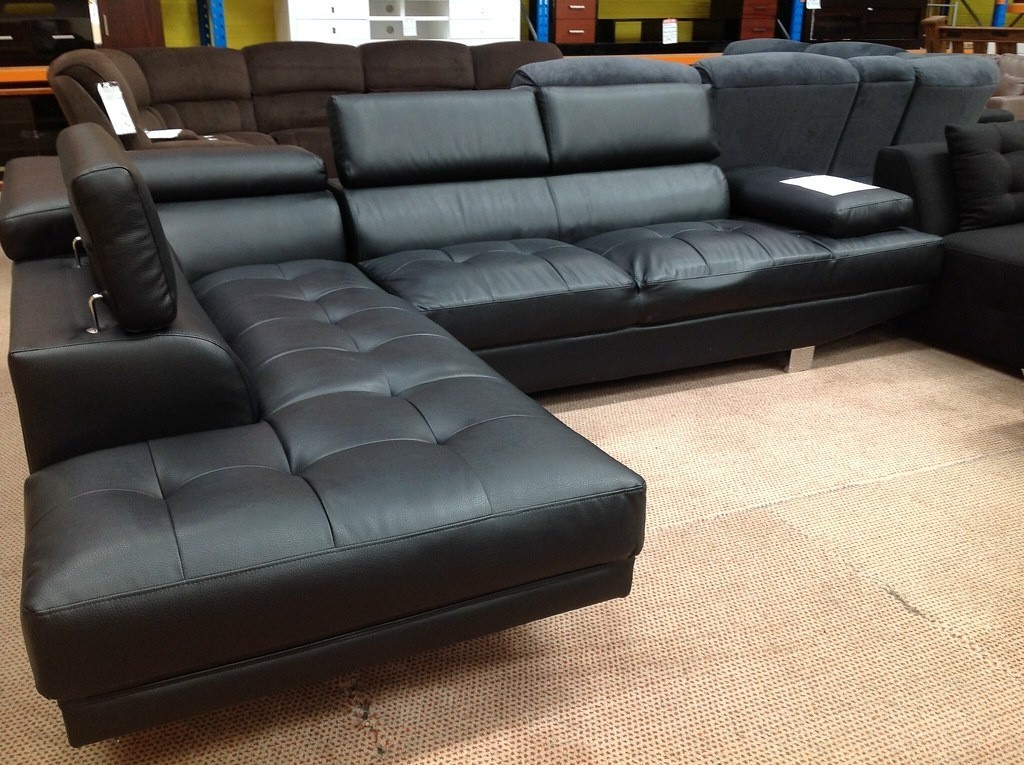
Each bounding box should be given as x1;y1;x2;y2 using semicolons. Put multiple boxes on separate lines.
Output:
273;0;521;47
706;0;780;41
549;0;598;44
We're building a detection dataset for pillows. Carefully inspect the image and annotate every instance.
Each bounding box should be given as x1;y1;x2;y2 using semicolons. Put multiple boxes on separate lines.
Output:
945;120;1024;233
756;174;914;235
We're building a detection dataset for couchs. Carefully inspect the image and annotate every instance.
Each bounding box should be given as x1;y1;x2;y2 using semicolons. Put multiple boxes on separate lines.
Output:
46;41;562;179
874;140;1024;381
973;53;1024;121
510;37;1002;184
0;84;947;748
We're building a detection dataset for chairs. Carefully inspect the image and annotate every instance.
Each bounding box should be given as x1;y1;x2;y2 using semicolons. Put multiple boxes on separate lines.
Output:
938;25;1024;55
921;16;946;53
